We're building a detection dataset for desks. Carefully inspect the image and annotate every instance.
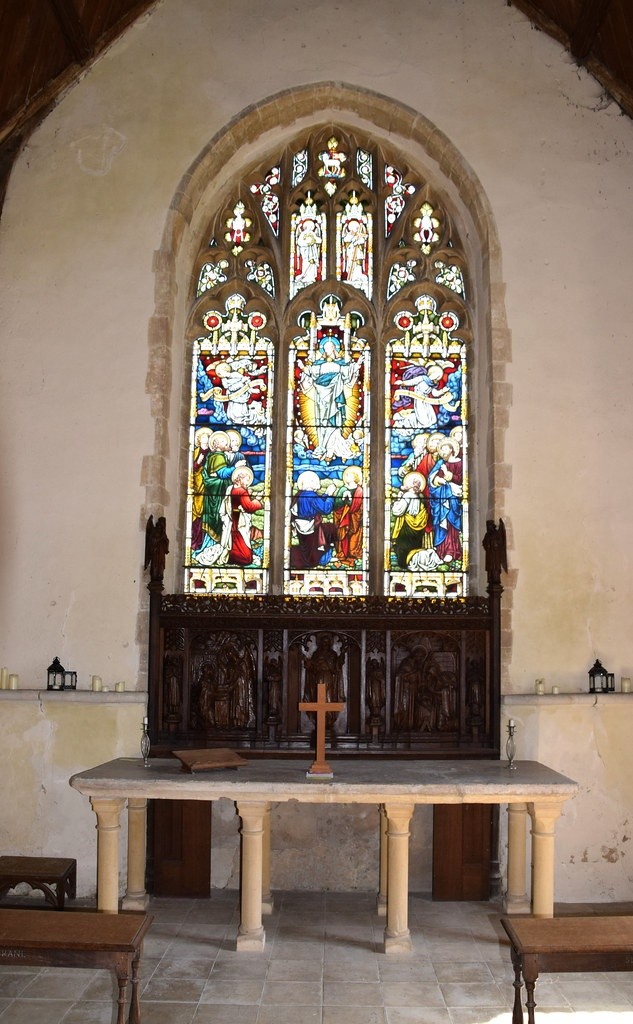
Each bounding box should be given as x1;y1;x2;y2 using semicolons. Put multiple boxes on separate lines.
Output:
501;917;633;1024
0;909;153;1024
68;756;581;955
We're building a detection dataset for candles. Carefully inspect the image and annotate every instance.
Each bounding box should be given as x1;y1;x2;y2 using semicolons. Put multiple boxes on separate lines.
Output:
92;675;102;692
116;681;125;692
9;674;19;690
534;678;545;695
509;719;514;727
143;716;147;724
552;686;559;694
102;686;109;692
621;677;631;693
1;666;8;689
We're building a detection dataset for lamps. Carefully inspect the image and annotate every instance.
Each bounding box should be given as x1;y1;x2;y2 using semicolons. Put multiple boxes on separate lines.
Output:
588;658;616;694
46;656;77;691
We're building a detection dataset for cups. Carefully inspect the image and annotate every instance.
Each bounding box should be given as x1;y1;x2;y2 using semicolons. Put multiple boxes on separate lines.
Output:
534;680;544;695
92;675;102;693
552;686;560;695
101;686;109;693
621;677;631;693
9;674;19;690
1;668;8;690
115;682;126;693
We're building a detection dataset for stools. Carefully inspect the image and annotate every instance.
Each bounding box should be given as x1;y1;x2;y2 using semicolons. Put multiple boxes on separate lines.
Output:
0;855;78;911
